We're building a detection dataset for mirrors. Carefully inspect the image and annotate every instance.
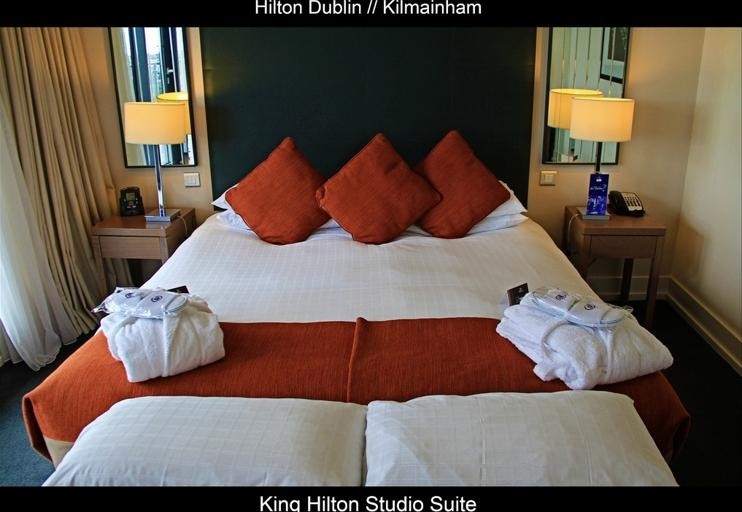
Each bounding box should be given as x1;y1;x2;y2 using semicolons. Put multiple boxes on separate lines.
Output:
106;26;198;171
541;27;633;170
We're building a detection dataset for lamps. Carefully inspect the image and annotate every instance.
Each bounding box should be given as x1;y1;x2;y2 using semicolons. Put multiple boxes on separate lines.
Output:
568;93;636;222
121;97;189;224
152;92;193;165
545;84;602;168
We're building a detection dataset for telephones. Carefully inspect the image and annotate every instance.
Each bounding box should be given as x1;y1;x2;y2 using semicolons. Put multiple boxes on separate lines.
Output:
608;191;643;216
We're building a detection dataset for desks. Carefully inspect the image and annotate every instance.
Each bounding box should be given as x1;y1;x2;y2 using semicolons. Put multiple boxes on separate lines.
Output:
89;203;199;311
560;200;668;332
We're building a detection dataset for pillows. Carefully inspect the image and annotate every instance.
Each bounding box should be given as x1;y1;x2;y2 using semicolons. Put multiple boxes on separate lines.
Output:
34;393;366;494
315;132;440;246
204;177;344;229
405;212;528;235
353;389;678;490
408;128;511;240
215;205;351;237
410;175;530;219
220;133;329;246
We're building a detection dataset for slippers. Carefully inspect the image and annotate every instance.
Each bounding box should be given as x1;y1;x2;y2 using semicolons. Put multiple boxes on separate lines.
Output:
531;288;626;328
102;288;187;319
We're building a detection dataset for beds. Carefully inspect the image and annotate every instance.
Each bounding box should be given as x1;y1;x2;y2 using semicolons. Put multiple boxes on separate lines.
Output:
15;25;689;478
